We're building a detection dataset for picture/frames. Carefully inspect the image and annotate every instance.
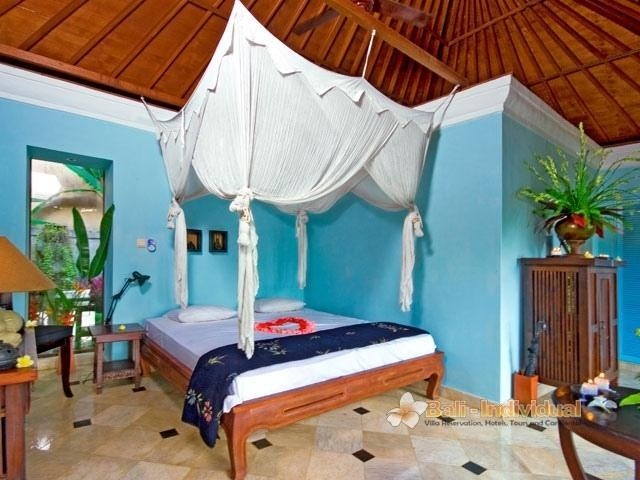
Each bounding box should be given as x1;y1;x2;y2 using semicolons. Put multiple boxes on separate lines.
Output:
209;230;229;253
186;228;203;252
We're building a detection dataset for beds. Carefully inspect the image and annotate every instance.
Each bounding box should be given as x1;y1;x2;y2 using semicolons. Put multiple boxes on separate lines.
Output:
139;306;445;479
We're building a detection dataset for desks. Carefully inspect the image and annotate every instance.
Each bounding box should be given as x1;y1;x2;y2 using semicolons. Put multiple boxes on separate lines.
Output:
18;325;74;398
0;366;38;480
551;383;639;480
74;305;100;350
88;322;149;389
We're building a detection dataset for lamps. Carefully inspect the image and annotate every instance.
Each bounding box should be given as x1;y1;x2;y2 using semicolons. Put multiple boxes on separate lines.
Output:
103;270;151;328
0;234;59;310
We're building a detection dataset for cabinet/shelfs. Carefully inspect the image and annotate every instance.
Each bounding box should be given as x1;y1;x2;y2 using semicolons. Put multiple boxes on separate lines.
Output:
518;255;627;404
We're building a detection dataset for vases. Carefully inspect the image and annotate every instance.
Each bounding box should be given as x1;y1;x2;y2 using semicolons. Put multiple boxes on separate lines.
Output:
555;217;598;255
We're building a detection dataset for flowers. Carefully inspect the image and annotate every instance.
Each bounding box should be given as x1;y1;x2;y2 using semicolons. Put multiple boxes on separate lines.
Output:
512;120;640;241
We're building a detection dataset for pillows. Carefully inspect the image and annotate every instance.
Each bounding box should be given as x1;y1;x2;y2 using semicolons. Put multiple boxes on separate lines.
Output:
254;298;308;314
164;305;238;324
